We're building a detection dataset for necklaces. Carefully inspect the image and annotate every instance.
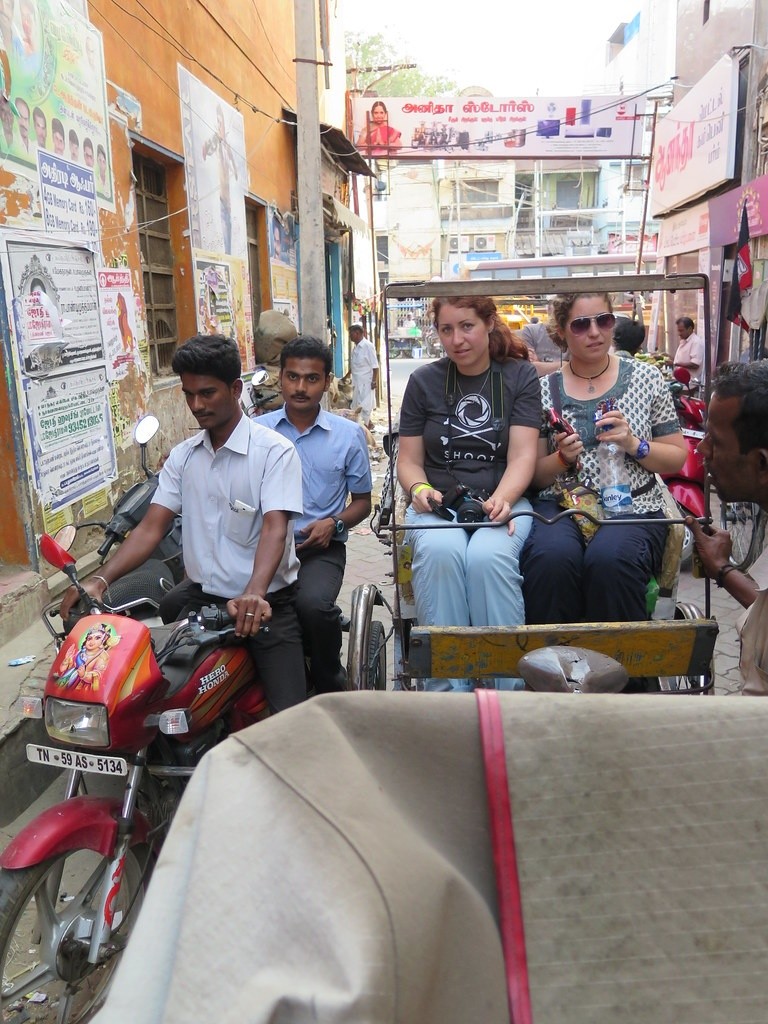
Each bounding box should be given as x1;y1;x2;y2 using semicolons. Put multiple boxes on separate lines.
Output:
570;353;610;394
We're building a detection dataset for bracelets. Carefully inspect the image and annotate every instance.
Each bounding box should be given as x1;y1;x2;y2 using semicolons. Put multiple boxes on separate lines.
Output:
717;565;736;588
558;450;579;473
410;482;433;502
673;362;676;366
94;576;111;603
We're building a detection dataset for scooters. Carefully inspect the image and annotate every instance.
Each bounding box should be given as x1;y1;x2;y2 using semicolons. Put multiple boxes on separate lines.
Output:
423;325;443;359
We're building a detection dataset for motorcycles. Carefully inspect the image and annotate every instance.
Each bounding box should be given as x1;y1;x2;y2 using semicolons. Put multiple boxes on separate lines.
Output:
0;536;313;1024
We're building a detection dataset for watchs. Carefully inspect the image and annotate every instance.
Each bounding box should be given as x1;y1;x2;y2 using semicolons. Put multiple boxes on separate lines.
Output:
331;515;345;535
626;438;650;459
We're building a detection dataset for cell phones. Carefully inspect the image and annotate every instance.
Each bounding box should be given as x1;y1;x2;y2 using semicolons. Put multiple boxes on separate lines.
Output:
234;500;255;512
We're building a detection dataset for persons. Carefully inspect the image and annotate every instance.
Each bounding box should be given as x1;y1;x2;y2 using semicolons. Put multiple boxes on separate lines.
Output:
398;297;542;692
686;360;768;696
356;101;401;155
404;315;416;327
665;317;705;395
61;335;373;713
521;293;687;694
340;325;379;428
0;0;40;71
271;218;282;258
521;298;646;379
0;94;108;187
31;278;47;308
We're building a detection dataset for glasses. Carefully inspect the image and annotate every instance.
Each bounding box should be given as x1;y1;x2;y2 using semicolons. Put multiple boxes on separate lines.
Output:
564;312;619;336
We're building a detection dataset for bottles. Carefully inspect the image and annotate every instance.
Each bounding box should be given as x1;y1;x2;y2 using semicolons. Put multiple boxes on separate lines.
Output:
597;405;633;512
412;123;456;149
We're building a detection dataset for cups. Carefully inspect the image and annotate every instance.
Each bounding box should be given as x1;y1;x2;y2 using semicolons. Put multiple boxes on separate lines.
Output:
513;130;526;146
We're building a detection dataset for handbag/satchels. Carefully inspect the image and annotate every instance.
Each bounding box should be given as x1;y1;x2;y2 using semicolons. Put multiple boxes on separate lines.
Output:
555;483;605;547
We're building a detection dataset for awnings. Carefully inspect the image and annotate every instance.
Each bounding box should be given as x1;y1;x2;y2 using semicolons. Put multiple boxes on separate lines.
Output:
323;192;366;237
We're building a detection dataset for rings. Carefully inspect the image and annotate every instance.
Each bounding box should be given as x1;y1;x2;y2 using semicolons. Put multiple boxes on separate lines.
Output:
246;613;255;617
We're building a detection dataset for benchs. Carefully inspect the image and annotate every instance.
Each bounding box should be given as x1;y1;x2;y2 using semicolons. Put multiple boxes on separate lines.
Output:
377;421;685;600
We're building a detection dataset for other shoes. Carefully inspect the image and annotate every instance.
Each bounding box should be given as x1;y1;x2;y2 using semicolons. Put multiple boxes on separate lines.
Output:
364;424;375;433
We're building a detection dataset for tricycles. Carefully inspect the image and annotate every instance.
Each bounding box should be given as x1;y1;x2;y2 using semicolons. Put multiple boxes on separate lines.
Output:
345;276;722;695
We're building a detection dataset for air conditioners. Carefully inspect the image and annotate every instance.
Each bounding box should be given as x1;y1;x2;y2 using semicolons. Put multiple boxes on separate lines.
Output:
448;236;469;253
473;235;495;251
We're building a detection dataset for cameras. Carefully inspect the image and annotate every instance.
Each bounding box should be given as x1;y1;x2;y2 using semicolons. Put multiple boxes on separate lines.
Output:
546;408;574;438
441;483;489;530
